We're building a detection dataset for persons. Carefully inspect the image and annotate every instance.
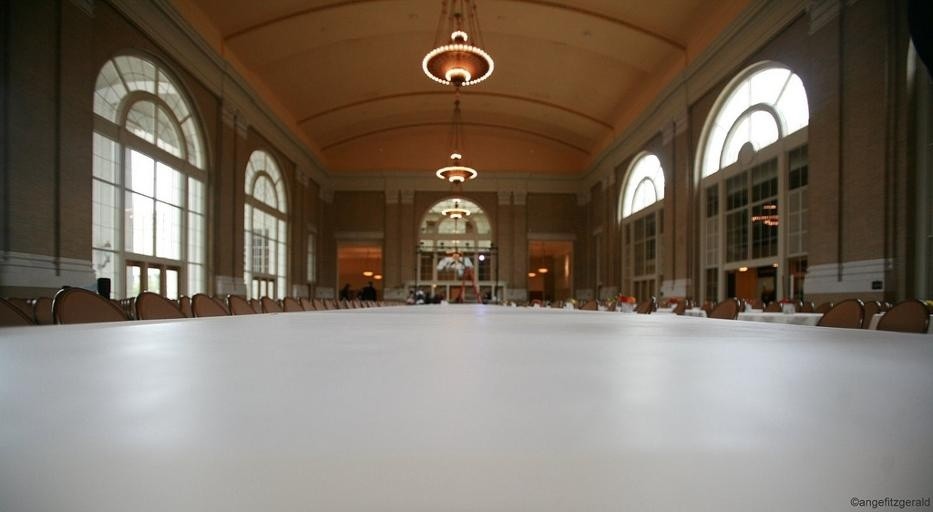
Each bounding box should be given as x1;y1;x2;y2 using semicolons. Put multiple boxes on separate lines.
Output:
404;286;443;304
760;284;770;306
339;282;350;301
361;279;379;301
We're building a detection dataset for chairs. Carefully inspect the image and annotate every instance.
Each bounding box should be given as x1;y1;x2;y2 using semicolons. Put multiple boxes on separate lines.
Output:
0;284;413;328
503;292;933;338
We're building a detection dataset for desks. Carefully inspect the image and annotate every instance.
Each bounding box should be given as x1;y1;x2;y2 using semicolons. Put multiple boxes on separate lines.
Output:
2;299;932;512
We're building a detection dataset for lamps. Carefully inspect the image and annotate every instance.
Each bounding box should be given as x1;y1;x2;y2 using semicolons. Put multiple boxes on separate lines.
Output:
435;99;480;184
442;196;473;220
419;1;496;88
